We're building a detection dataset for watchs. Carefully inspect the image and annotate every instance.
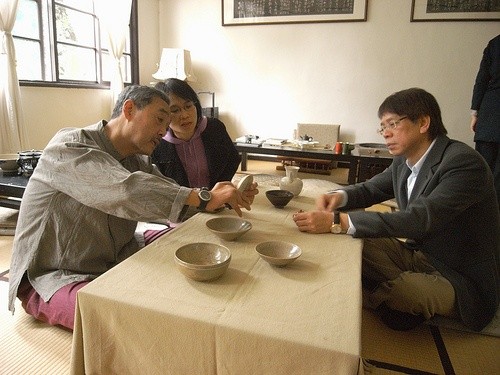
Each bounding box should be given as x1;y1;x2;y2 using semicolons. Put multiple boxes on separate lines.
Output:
330;212;342;234
196;186;212;212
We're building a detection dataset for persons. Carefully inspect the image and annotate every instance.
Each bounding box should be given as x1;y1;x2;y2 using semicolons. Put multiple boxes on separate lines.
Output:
7;85;260;331
147;78;242;192
292;88;500;332
470;34;500;200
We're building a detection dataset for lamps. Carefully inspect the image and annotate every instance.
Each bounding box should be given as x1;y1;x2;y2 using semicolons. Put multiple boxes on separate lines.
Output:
152;47;194;83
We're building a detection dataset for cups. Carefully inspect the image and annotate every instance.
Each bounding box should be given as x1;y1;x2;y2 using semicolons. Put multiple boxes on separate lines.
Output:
336;141;342;154
245;134;251;143
342;142;349;155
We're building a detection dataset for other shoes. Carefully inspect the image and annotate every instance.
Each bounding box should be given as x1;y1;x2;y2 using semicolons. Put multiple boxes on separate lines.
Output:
382;310;439;331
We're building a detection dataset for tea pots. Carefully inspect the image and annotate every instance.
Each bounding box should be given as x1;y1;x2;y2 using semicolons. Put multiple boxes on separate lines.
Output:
299;134;313;142
280;166;303;198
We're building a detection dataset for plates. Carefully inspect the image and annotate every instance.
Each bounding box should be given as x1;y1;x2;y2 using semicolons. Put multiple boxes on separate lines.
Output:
236;174;255;206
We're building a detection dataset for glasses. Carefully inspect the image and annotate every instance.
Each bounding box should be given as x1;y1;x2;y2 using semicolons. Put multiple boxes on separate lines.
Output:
376;112;425;137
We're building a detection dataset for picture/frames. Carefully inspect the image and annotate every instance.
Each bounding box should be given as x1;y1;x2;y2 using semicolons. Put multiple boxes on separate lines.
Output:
221;0;368;26
410;0;500;23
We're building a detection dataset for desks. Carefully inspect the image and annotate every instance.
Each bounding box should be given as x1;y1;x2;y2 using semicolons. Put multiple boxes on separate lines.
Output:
0;169;29;236
71;174;365;375
234;142;393;184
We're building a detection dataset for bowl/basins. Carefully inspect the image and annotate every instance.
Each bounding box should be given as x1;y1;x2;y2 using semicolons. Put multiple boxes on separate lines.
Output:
0;158;21;172
255;240;302;268
17;150;43;179
174;242;231;282
205;217;252;241
265;189;294;209
